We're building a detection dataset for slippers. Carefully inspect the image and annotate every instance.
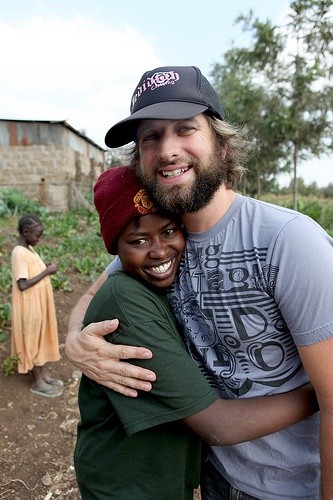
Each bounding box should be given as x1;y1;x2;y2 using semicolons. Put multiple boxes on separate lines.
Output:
32;379;64;388
30;384;63;398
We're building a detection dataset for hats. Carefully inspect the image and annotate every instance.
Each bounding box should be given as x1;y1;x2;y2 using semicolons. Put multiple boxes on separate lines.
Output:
105;66;224;148
93;166;171;255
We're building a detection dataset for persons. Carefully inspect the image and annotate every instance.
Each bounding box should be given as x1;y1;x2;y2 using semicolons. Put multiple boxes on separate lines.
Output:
64;65;332;500
9;214;66;398
71;164;322;500
